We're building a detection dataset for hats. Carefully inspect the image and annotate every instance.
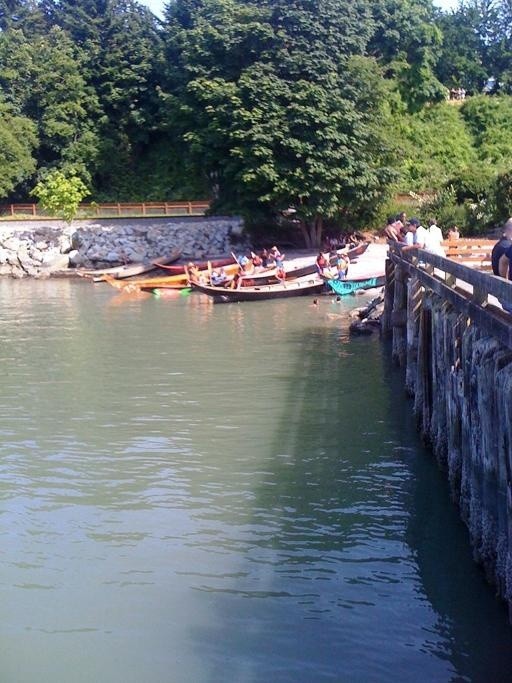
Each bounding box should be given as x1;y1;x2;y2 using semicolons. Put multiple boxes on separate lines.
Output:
405;218;419;225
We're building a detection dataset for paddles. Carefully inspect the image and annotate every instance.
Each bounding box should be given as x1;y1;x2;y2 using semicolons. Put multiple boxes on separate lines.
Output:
207;261;213;286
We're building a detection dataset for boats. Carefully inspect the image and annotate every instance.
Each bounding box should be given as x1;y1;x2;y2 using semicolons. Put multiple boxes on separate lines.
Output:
102;259;253;292
184;241;371;284
315;263;386;296
152;255;253;276
76;248;182;282
187;271;340;302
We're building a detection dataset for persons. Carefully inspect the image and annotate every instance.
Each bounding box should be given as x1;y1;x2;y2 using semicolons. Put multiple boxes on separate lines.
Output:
315;251;330;278
336;297;342;303
498;244;512;312
491;217;512;276
333;253;347;280
447;226;463;257
384;211;446;280
187;245;288;289
309;299;319;307
329;231;358;250
342;253;350;278
449;86;467;100
